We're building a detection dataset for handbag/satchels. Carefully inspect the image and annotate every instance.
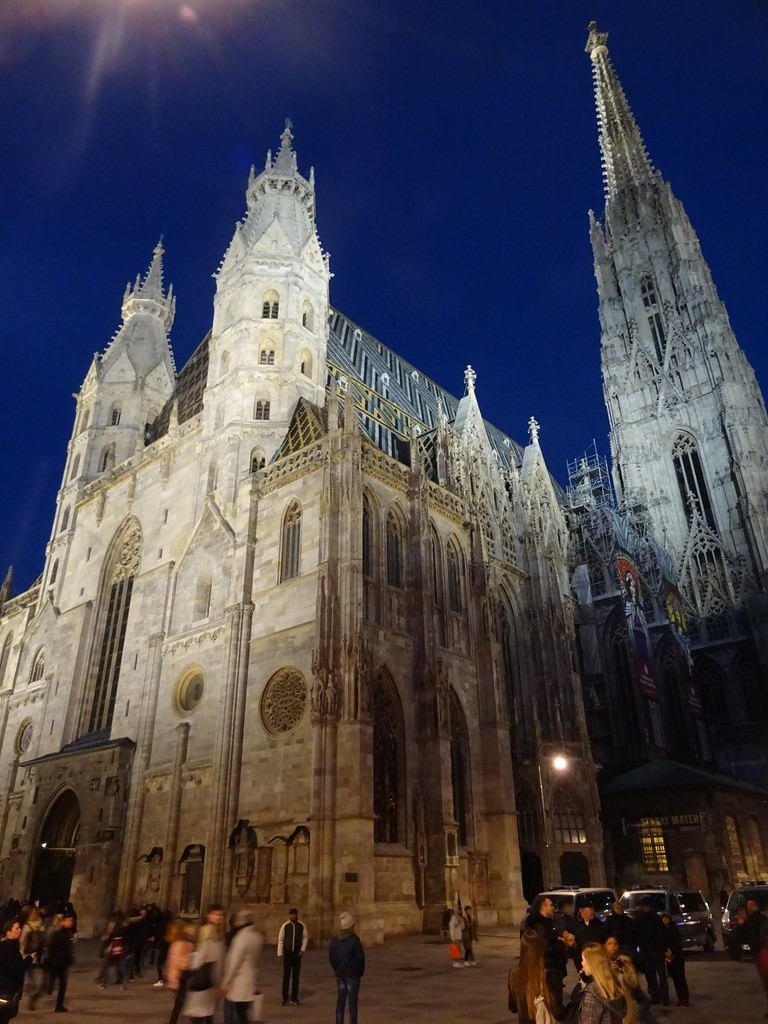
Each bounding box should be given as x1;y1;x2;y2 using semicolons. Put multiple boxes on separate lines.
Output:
0;992;20;1018
448;943;462;959
183;963;213;992
534;995;560;1024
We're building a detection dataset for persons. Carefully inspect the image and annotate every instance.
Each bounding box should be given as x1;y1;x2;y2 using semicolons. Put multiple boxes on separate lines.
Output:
329;912;365;1024
277;908;309;1007
0;897;78;1024
97;903;265;1024
719;885;729;908
508;895;690;1024
625;572;652;664
734;900;768;1018
442;906;479;968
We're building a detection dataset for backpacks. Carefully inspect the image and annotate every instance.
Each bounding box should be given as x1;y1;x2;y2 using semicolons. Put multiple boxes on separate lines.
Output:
23;922;48;955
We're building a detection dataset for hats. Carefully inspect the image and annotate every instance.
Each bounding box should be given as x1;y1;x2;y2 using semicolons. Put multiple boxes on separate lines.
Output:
235;910;252;923
339;911;357;930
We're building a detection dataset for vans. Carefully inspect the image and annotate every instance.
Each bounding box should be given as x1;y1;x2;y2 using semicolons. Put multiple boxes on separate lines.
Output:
721;880;768;960
616;889;718;952
518;886;619;944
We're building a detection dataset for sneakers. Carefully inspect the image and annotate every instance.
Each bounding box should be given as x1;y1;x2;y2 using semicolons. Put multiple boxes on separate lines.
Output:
55;1006;68;1013
281;1001;287;1006
127;977;135;983
152;980;164;987
289;1000;300;1005
675;1000;691;1011
463;961;478;967
650;998;671;1010
453;962;465;968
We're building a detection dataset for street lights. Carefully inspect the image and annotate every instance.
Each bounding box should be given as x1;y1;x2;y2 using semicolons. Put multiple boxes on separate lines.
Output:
535;755;568;889
